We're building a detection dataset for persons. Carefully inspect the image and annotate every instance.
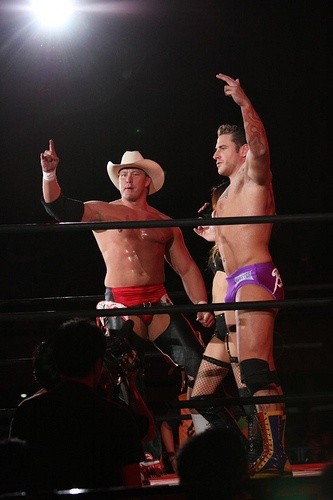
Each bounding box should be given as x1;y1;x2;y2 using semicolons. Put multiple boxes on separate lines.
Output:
43;139;214;381
192;182;264;466
212;73;294;479
0;318;333;500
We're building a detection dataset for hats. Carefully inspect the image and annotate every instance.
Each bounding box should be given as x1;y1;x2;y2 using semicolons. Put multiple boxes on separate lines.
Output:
106;150;164;196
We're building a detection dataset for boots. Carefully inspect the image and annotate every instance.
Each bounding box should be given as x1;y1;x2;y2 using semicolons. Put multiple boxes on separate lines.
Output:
185;395;251;461
247;405;270;460
250;412;292;480
